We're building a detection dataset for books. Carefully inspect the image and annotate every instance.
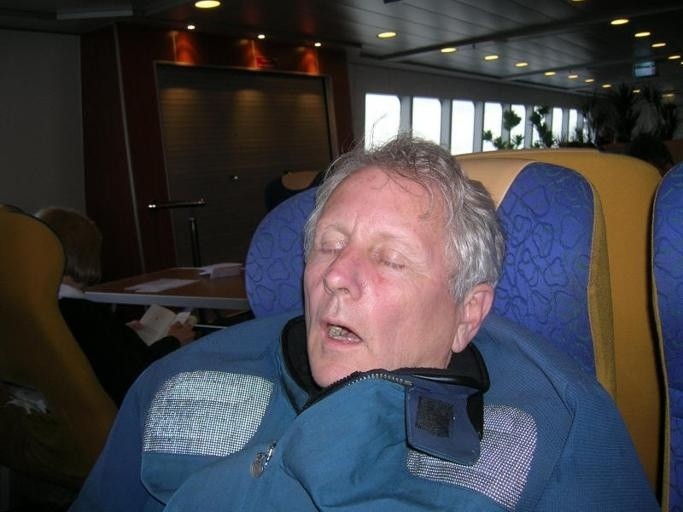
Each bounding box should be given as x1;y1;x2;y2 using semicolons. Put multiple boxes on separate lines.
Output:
134;302;198;349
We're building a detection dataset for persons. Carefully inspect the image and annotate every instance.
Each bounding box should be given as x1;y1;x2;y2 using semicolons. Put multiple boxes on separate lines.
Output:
67;134;661;512
33;207;196;417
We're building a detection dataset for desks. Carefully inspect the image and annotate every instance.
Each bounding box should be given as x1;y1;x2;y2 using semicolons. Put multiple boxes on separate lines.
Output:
83;263;254;337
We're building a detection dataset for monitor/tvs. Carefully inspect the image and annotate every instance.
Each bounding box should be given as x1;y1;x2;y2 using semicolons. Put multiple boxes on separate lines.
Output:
631;60;659;78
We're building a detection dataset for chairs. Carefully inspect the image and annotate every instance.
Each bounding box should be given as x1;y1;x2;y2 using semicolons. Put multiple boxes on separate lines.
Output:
454;148;660;499
647;160;683;512
244;154;615;409
0;203;124;512
